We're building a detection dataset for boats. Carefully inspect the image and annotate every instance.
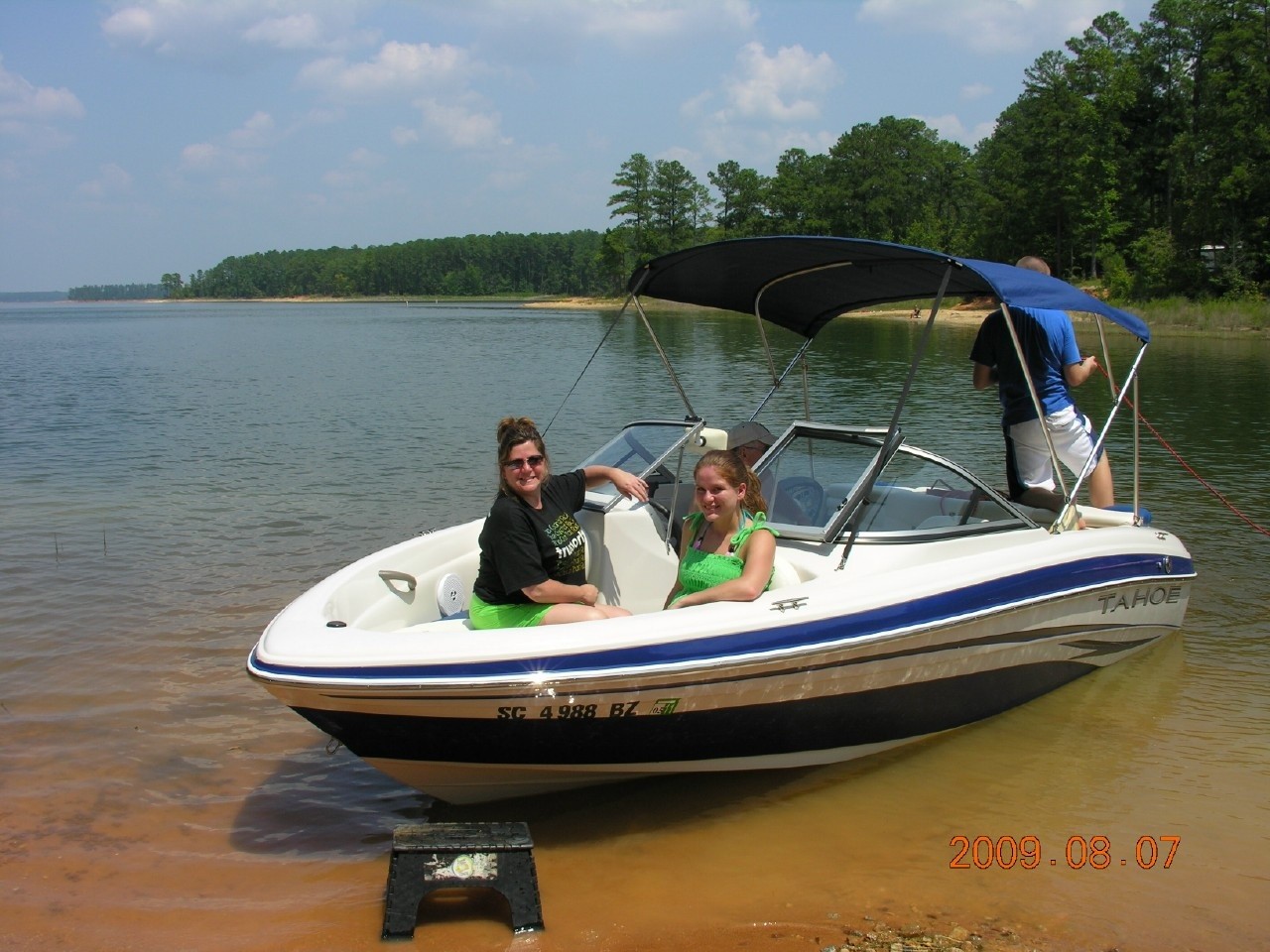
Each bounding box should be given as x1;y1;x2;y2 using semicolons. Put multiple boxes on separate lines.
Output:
247;234;1198;808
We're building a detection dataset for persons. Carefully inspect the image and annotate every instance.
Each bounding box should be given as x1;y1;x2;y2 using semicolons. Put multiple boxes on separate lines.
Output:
662;449;780;610
969;255;1114;530
469;417;649;629
910;305;920;318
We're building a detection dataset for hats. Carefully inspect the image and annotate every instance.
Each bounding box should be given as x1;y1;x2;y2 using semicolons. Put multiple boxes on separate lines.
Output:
725;421;782;450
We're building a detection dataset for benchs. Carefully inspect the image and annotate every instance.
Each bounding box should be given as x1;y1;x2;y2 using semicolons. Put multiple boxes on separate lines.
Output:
818;484;1055;557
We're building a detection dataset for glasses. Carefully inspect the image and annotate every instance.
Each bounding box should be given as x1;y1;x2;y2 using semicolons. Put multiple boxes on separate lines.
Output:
502;454;545;470
743;444;770;455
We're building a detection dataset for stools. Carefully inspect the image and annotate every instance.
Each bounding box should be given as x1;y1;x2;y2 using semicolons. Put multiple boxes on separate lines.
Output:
381;823;544;939
